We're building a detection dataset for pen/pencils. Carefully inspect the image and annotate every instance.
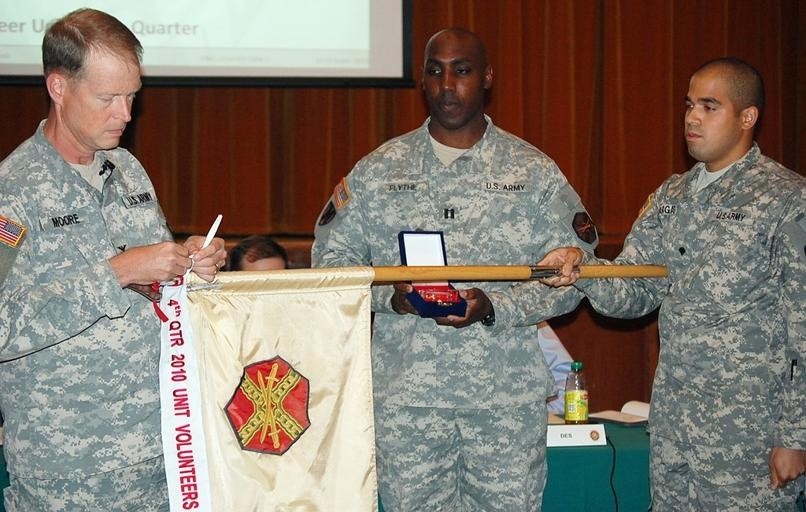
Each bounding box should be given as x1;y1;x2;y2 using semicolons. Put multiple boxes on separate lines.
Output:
201;214;224;250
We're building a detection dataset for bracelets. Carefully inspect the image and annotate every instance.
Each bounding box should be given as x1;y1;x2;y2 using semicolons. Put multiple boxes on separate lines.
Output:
481;314;495;326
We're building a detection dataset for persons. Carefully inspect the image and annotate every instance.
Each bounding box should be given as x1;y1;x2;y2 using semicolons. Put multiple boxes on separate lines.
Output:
230;235;288;271
536;57;806;512
312;28;599;511
538;320;580;415
0;9;228;512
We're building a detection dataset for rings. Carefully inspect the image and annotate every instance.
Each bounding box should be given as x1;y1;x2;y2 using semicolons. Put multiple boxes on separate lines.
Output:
214;264;219;275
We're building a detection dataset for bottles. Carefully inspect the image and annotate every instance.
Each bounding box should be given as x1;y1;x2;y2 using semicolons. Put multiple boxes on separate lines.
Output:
563;361;592;426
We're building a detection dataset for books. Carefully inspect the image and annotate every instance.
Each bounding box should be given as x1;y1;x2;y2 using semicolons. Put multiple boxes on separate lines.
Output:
588;400;650;426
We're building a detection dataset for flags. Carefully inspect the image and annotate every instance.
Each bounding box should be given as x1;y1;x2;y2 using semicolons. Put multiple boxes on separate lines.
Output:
159;268;379;512
